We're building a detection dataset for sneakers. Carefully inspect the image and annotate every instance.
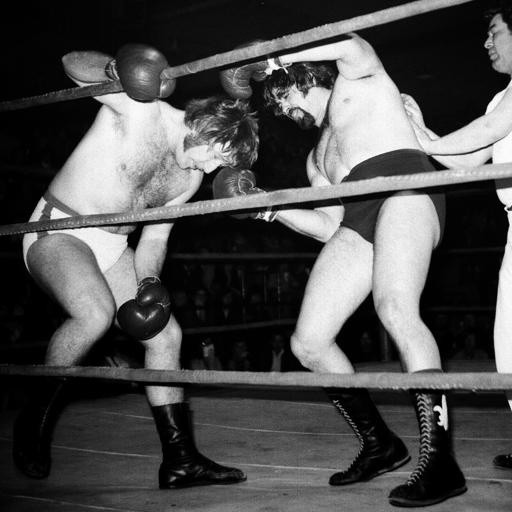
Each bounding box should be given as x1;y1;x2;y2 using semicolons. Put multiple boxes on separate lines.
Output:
491;454;511;471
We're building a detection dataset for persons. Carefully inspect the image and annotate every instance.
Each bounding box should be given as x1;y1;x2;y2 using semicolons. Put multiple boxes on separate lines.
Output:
422;309;494;369
168;245;310;372
400;8;512;470
0;281;68;345
9;46;259;490
213;31;468;507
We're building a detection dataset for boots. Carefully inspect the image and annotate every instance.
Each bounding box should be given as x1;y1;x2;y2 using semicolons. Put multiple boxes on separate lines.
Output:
9;377;70;481
150;400;248;491
387;367;468;509
322;388;412;487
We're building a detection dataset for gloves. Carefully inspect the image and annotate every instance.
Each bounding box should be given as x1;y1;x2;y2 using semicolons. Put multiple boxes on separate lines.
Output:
104;43;178;103
218;37;293;102
115;275;172;342
210;165;280;225
